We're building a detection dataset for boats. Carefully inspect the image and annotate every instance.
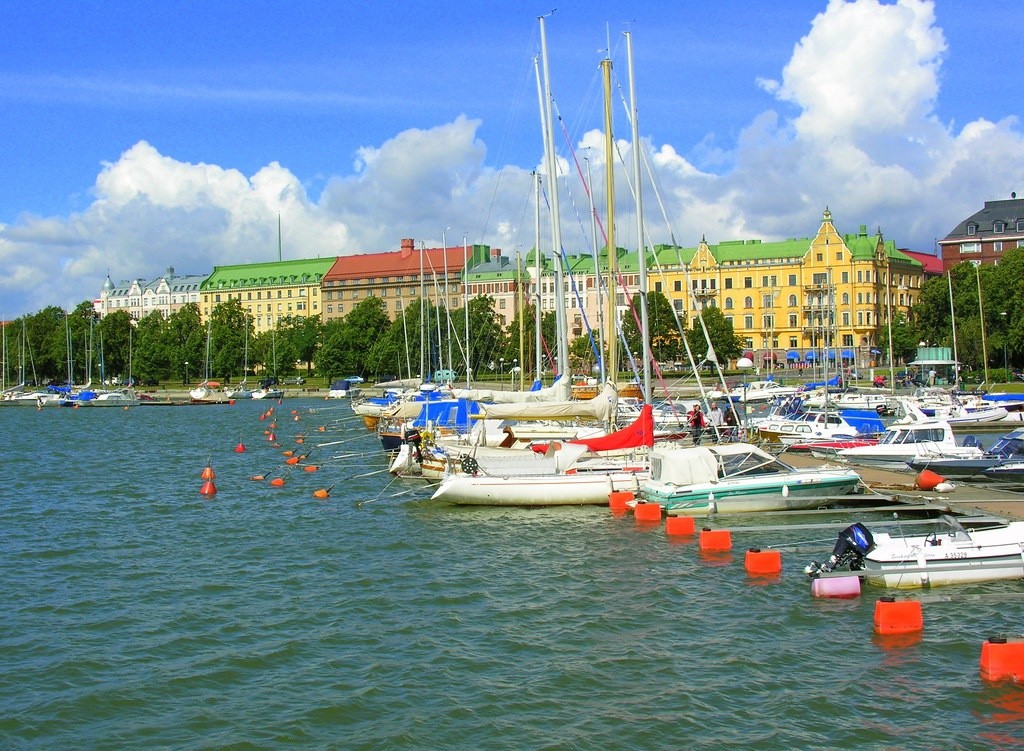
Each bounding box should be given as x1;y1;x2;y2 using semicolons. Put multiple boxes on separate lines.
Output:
803;519;1024;590
624;441;864;517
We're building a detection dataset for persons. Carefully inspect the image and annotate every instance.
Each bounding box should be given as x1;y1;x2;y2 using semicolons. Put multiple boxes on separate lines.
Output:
755;365;760;376
688;402;741;445
798;367;803;377
872;368;936;389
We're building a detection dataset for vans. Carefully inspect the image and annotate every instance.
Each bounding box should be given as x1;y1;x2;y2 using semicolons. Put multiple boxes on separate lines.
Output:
120;378;139;387
434;369;460;383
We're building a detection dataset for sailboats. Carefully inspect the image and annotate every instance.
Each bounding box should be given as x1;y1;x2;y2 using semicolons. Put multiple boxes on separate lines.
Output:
251;314;284;399
0;313;141;407
223;314;253;400
189;318;231;403
349;1;1023;507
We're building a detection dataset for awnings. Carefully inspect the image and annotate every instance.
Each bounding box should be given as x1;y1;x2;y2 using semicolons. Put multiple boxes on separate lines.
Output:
744;350;856;361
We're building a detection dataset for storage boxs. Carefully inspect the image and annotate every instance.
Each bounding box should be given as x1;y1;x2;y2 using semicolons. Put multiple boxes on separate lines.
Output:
936;378;947;385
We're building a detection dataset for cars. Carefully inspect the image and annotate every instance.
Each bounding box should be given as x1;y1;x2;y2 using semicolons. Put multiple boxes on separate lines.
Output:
344;375;365;385
373;374;397;385
138;378;159;388
282;376;306;385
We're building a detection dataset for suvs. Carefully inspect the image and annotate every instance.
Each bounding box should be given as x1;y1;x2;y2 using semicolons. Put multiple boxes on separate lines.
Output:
104;376;123;386
